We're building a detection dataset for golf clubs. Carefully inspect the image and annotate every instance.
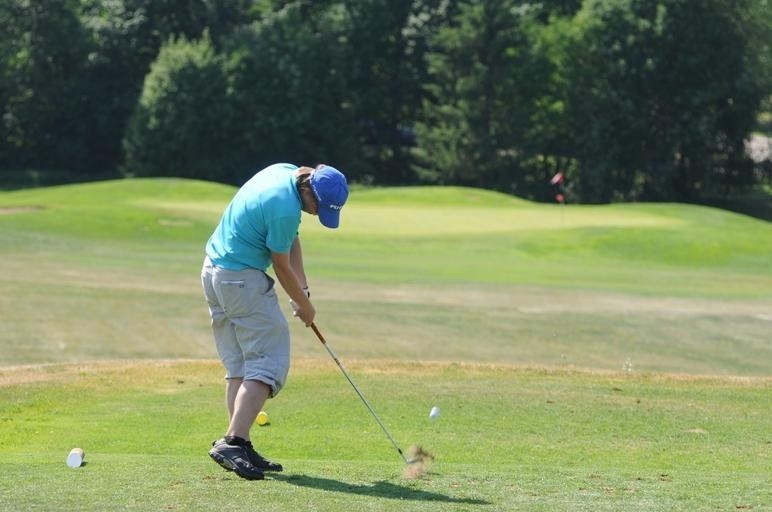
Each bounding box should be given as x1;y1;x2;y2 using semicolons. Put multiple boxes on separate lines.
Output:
289;297;423;467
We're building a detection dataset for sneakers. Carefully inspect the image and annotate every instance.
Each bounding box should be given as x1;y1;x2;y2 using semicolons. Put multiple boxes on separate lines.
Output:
207;438;264;481
224;436;285;474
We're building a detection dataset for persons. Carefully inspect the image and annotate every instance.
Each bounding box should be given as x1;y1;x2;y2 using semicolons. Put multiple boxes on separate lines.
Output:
201;163;350;480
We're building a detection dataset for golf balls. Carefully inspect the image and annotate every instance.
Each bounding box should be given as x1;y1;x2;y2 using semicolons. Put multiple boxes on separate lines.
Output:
430;407;440;418
256;411;268;425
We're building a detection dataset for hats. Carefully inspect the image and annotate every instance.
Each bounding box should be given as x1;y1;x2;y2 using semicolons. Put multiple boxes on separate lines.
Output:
309;164;351;229
